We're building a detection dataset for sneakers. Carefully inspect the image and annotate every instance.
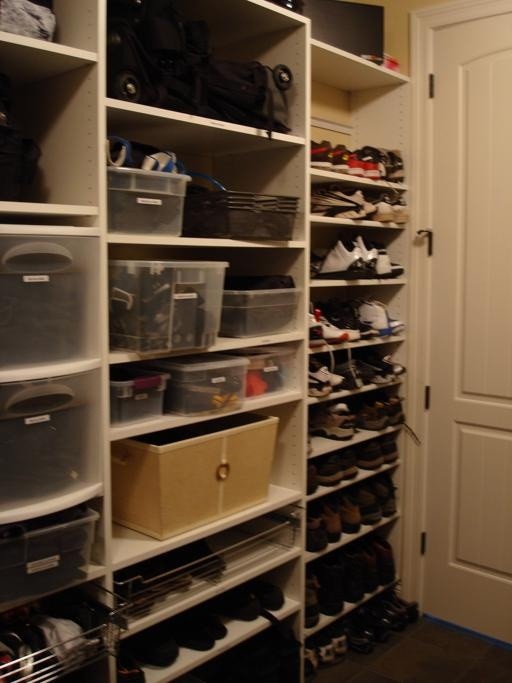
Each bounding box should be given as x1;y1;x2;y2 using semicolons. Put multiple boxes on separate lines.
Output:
311;140;406;182
307;393;405;440
310;236;405;280
311;186;409;225
308;295;406;347
308;352;407;397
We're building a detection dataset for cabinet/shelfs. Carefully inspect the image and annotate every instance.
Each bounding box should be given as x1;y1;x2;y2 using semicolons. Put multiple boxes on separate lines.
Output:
301;30;419;669
99;0;312;682
0;0;125;683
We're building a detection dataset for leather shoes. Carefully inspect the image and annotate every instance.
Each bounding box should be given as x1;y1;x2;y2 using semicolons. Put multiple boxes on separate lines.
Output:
117;573;284;666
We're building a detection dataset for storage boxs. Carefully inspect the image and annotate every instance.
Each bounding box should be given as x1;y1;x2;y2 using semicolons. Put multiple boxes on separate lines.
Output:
242;347;295;400
103;161;194;238
108;258;226;353
215;282;304;338
290;0;390;66
0;254;85;366
169;355;246;419
114;413;278;545
182;183;300;245
1;502;105;599
1;396;93;507
112;373;169;424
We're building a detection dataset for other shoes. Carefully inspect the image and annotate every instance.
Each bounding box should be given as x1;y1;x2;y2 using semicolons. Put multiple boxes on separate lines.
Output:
307;477;396;553
305;536;395;628
305;596;418;671
307;438;399;495
